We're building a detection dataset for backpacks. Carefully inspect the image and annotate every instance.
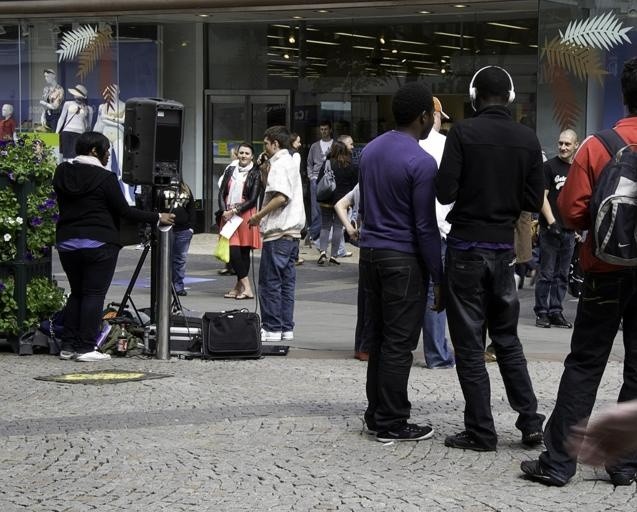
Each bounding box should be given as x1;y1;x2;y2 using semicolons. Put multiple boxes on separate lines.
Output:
588;128;637;266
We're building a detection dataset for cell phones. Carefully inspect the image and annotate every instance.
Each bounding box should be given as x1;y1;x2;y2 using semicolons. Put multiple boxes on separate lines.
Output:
116;186;183;322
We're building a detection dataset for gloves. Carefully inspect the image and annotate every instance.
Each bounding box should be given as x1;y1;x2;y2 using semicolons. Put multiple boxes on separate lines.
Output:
515;259;536;276
431;284;445;314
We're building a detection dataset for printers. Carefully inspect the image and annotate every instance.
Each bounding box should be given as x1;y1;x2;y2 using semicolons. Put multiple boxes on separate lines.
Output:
470;105;478;111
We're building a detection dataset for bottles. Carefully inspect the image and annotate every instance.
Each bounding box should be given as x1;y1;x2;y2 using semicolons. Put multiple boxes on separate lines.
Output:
117;323;128;357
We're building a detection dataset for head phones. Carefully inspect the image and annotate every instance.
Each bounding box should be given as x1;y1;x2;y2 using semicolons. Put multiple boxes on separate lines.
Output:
469;66;516;104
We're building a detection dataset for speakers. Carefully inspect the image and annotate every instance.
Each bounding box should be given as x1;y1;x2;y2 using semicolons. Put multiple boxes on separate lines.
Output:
122;97;185;187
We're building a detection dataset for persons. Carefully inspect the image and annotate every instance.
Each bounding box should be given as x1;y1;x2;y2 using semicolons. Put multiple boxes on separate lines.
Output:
40;68;64;132
1;104;17;149
56;85;93;157
51;132;177;362
98;84;128;173
521;66;637;485
360;67;546;452
216;120;369;361
154;185;196;296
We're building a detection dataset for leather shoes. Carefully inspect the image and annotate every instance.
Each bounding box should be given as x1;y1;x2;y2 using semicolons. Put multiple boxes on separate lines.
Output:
549;311;572;328
536;313;551;328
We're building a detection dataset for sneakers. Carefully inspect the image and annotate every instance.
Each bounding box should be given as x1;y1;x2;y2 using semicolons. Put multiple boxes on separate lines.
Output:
295;239;352;266
611;471;637;485
367;421;434;441
60;350;78;359
177;290;187;296
219;263;236;274
260;327;294;342
521;430;543;444
445;430;495;452
354;351;497;370
521;460;573;486
76;351;111;361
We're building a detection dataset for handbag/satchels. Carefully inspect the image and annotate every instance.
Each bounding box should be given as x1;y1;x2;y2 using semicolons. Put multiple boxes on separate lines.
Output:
41;312;63;338
201;310;262;359
316;171;337;200
569;241;584;297
215;235;230;263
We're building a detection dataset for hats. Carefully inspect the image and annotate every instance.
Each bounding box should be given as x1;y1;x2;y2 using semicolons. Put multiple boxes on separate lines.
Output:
433;97;450;120
68;84;87;98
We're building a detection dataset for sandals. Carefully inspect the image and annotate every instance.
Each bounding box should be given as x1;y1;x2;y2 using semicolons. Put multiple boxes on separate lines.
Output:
224;291;254;299
